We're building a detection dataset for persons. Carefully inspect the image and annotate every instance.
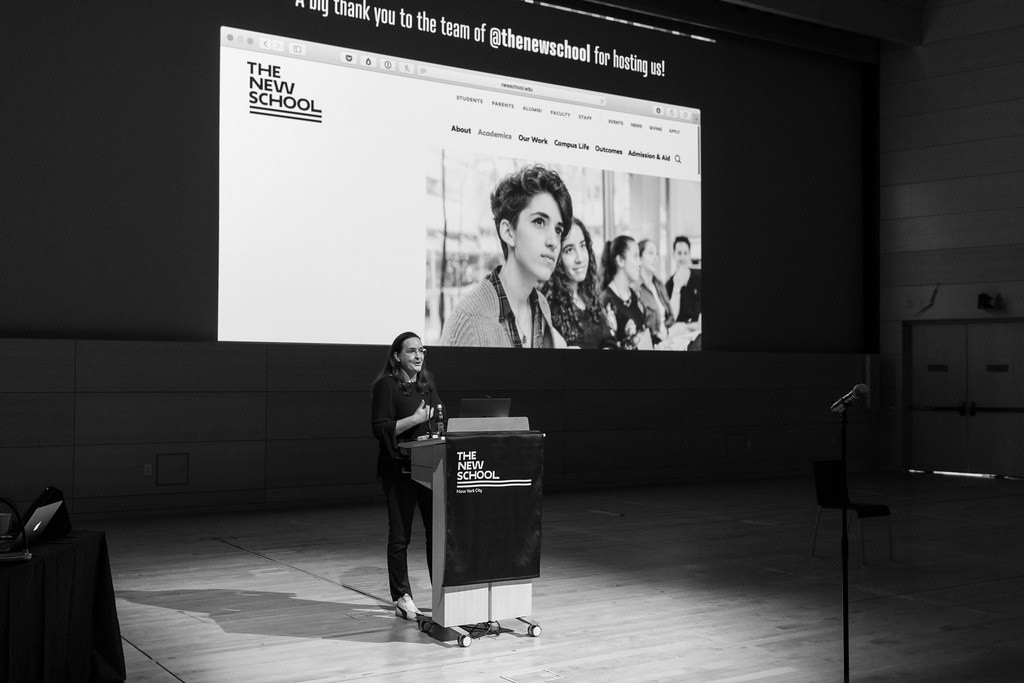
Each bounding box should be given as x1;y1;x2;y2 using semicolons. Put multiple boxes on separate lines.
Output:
534;216;618;350
638;239;677;351
597;235;664;350
368;331;449;622
439;163;574;349
664;236;701;351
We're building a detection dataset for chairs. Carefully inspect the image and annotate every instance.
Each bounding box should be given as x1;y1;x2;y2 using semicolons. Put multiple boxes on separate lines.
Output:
810;459;894;560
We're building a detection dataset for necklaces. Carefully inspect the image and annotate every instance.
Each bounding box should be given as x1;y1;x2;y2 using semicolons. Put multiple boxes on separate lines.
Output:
613;280;631;304
514;299;530;344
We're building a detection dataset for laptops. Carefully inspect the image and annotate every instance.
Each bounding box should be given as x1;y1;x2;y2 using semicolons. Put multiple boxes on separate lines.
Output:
458;398;511;418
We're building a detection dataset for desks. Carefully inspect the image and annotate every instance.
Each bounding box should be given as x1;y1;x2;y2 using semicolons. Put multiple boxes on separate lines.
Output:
0;526;126;683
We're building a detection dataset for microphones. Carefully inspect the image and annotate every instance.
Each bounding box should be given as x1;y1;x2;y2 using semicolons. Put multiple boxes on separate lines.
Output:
427;388;433;441
